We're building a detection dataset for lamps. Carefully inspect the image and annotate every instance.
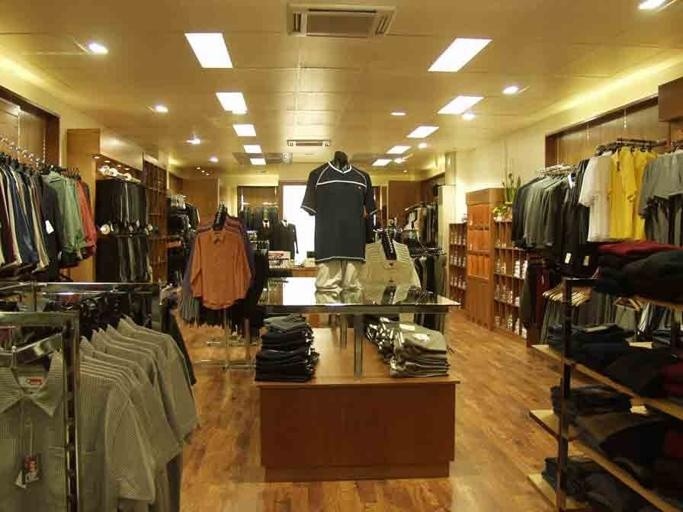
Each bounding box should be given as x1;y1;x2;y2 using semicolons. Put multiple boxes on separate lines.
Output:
636;0;671;17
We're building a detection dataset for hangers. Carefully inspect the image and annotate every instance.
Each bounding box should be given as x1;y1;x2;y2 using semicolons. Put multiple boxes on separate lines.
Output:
593;139;658;154
0;135;89;180
2;280;164;509
210;205;228;232
100;161;140;186
538;167;577;181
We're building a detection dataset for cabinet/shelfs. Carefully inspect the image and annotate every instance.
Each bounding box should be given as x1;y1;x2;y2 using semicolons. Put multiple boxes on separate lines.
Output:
254;278;462;479
448;221;466;319
466;188;516;330
491;220;525;346
525;248;683;507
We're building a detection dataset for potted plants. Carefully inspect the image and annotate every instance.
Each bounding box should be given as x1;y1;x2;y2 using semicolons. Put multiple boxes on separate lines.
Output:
500;172;520;220
491;202;507;222
